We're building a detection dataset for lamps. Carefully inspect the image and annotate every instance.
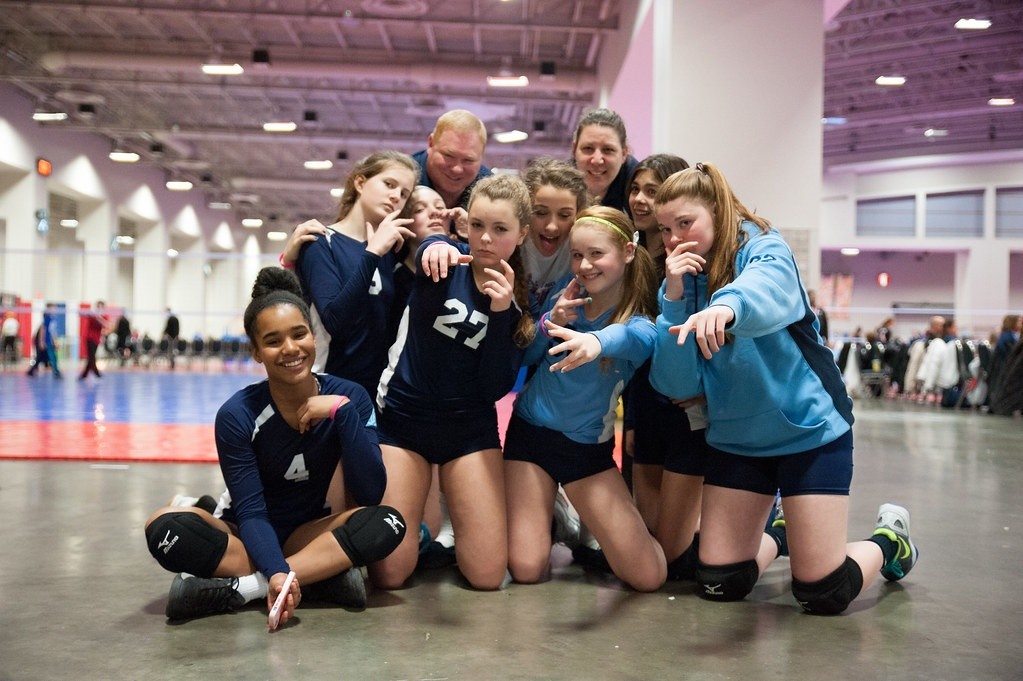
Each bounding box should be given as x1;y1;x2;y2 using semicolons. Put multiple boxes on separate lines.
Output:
31;46;352;240
488;56;558;144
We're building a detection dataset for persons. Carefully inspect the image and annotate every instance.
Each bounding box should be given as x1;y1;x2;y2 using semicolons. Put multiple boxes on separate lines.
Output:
836;314;1023;418
144;266;406;630
114;308;132;365
145;108;918;631
806;289;828;346
648;161;919;617
0;311;20;362
25;304;65;380
77;302;112;381
162;308;179;369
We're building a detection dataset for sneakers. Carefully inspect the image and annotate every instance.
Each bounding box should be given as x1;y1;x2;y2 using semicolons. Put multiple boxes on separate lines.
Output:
579;516;601;550
302;566;366;607
772;517;786;527
165;572;246;619
873;503;919;580
553;491;582;546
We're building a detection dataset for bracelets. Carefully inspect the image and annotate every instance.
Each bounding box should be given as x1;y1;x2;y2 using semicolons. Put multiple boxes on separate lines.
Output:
539;312;555;339
329;396;348;419
278;253;295;269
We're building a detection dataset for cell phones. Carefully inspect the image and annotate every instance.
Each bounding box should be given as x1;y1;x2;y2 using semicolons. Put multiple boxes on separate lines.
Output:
268;571;296;630
